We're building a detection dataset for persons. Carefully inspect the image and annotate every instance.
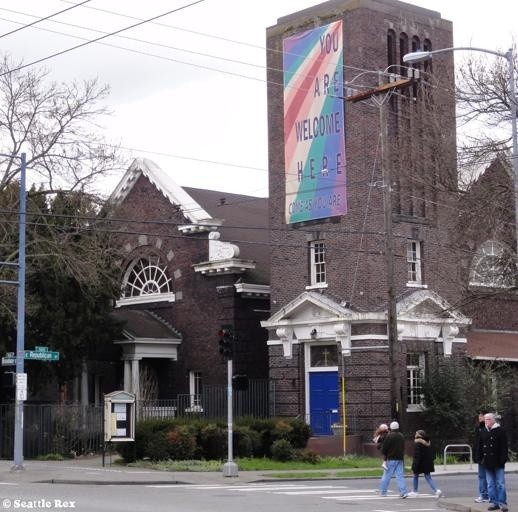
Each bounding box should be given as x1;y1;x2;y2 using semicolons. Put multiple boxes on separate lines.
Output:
409;429;442;498
375;423;390;470
480;412;509;511
472;409;489;503
378;421;409;498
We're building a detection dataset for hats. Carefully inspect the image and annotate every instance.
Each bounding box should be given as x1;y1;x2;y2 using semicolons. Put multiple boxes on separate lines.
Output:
390;421;400;430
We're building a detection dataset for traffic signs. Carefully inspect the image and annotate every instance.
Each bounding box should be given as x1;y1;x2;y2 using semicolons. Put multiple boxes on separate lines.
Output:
2;352;16;366
24;346;59;360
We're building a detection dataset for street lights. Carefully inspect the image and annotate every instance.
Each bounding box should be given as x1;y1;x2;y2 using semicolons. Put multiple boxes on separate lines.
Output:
402;46;517;243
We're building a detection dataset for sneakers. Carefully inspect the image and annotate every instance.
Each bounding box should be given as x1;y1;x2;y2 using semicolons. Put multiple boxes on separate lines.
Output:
474;495;509;512
376;488;442;500
381;464;388;471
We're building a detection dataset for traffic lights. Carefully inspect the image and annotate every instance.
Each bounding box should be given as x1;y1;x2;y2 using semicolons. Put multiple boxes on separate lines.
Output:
218;323;235;357
